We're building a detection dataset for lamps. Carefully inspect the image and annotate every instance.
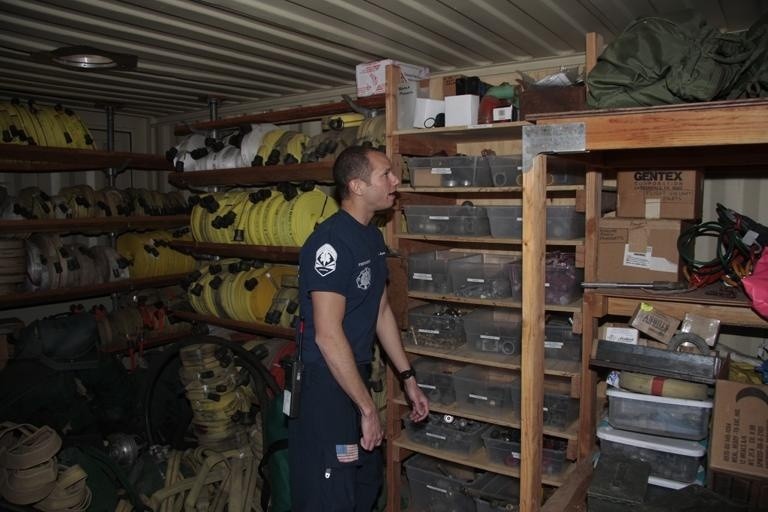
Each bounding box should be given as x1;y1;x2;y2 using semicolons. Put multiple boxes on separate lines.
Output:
34;45;138;73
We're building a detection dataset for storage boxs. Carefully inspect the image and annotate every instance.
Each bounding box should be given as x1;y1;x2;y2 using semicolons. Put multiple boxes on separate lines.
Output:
402;153;581;188
399;408;570;477
406;250;584;307
595;212;695;285
402;452;520;512
405;302;583;363
614;168;703;222
406;357;580;433
586;424;709;512
604;386;714;441
707;352;768;478
402;203;585;240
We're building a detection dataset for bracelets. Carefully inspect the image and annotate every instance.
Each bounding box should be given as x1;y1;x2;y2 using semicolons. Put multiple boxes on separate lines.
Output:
398;368;416;381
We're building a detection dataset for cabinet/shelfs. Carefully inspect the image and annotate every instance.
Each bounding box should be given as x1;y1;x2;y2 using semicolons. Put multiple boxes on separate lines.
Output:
176;95;387;442
518;98;767;511
386;32;602;512
1;144;202;373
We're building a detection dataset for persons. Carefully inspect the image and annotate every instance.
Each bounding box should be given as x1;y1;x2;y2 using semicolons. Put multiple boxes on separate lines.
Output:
284;144;430;512
